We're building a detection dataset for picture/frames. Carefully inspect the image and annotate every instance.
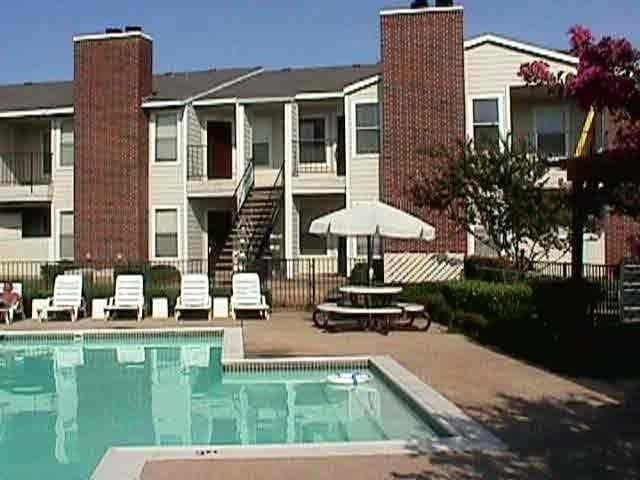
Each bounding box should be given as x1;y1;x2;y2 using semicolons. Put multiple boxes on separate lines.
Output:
339;284;402;331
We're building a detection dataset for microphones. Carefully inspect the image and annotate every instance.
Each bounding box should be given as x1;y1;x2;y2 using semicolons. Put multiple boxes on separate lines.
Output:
313;302;431;336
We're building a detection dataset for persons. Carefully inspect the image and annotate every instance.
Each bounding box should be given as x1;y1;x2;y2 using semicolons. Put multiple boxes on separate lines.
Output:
0;280;20;330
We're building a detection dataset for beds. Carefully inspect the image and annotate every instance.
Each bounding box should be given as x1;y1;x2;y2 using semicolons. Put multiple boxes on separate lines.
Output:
308;193;436;309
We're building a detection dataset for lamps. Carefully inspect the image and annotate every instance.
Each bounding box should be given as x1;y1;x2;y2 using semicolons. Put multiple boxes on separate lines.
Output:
36;275;87;328
0;282;26;327
55;347;85;369
180;344;210;367
116;346;145;367
229;273;270;321
104;275;145;328
174;274;212;325
